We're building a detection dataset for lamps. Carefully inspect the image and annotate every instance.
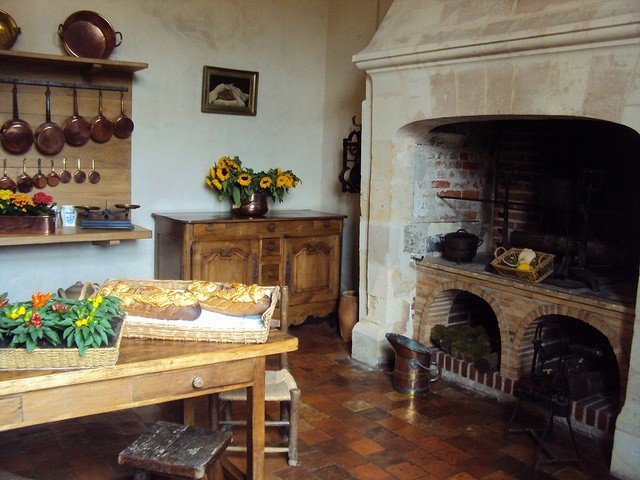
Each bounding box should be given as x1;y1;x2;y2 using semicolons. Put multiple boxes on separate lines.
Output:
209;286;302;467
504;319;583;472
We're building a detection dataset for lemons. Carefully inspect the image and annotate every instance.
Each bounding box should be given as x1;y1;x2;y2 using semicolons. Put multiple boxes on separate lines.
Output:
57;281;100;303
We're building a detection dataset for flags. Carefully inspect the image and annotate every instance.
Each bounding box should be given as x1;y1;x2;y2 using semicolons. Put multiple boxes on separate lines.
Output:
232;190;268;217
1;215;55;235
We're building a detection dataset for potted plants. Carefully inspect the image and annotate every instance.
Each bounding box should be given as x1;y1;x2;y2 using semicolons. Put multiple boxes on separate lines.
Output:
202;66;258;115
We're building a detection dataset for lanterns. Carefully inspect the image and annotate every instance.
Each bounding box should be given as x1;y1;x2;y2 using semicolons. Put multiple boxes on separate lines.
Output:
517;264;530;271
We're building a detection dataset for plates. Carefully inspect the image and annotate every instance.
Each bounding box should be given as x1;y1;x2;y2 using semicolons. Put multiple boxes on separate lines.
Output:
0;48;153;247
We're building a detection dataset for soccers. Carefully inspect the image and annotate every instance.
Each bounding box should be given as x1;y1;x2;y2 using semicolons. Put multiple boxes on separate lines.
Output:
518;249;536;264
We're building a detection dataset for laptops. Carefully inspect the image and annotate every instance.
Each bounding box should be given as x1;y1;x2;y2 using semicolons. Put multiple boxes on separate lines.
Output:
186;281;270;316
101;281;199;320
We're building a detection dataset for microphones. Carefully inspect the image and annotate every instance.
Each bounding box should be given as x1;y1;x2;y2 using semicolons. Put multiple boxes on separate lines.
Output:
118;420;234;480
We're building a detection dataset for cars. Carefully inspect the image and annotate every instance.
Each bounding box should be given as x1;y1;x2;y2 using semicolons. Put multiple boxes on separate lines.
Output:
60;205;78;227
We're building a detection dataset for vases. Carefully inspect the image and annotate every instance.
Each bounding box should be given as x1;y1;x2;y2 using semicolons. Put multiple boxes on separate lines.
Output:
28;192;53;216
10;193;36;215
0;291;127;356
205;156;303;209
0;188;14;216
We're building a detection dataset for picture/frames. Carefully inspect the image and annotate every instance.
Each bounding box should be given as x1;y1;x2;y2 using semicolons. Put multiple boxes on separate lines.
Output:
151;209;347;329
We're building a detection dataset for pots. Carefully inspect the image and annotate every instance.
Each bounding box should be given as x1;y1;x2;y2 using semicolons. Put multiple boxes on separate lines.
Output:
60;156;72;184
113;88;134;139
33;158;48;189
438;228;484;264
0;9;22;50
18;158;34;193
48;156;60;188
73;158;86;184
91;87;114;144
57;9;123;60
62;83;91;147
34;84;66;156
0;158;17;194
1;80;34;155
88;159;101;185
